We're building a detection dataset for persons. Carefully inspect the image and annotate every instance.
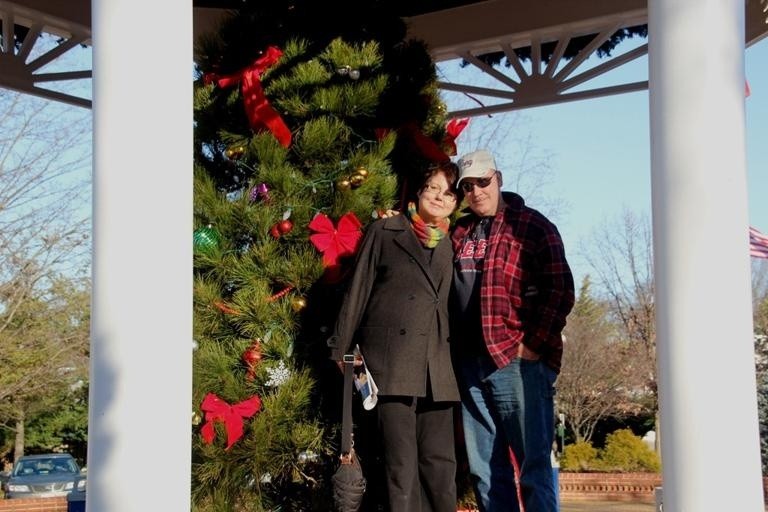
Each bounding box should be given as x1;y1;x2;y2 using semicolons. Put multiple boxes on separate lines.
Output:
380;150;575;511
327;161;458;512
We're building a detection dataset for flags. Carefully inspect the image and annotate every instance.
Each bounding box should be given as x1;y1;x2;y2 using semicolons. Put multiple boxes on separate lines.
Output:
750;226;768;258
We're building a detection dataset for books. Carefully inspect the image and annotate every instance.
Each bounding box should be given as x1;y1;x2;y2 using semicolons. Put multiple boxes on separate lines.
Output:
352;344;379;410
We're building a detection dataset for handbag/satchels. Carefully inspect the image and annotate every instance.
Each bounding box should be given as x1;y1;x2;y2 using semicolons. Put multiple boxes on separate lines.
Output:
332;444;369;511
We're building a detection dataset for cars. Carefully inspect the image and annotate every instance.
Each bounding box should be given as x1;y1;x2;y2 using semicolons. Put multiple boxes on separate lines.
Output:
4;453;81;499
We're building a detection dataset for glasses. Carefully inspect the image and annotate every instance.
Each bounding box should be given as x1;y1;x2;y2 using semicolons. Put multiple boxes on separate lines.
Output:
463;172;496;192
425;183;457;201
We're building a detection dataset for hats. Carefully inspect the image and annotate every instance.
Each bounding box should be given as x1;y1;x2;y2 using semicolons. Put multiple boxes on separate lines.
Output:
456;150;497;188
